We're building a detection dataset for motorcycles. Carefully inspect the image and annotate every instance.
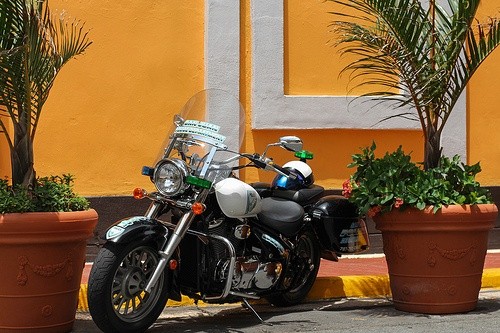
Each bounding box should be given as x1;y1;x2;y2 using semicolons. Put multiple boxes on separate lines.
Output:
87;88;359;333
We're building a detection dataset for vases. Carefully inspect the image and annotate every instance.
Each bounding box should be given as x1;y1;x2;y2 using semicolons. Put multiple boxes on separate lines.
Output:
374;203;499;314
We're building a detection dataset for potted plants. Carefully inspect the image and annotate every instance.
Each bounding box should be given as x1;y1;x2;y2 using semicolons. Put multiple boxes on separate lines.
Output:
0;0;99;332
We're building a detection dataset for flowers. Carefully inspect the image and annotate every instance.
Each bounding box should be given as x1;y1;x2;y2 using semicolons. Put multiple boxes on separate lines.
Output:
340;143;491;219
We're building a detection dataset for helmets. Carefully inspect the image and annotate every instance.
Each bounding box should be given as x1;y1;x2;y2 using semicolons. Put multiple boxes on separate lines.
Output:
272;161;315;191
